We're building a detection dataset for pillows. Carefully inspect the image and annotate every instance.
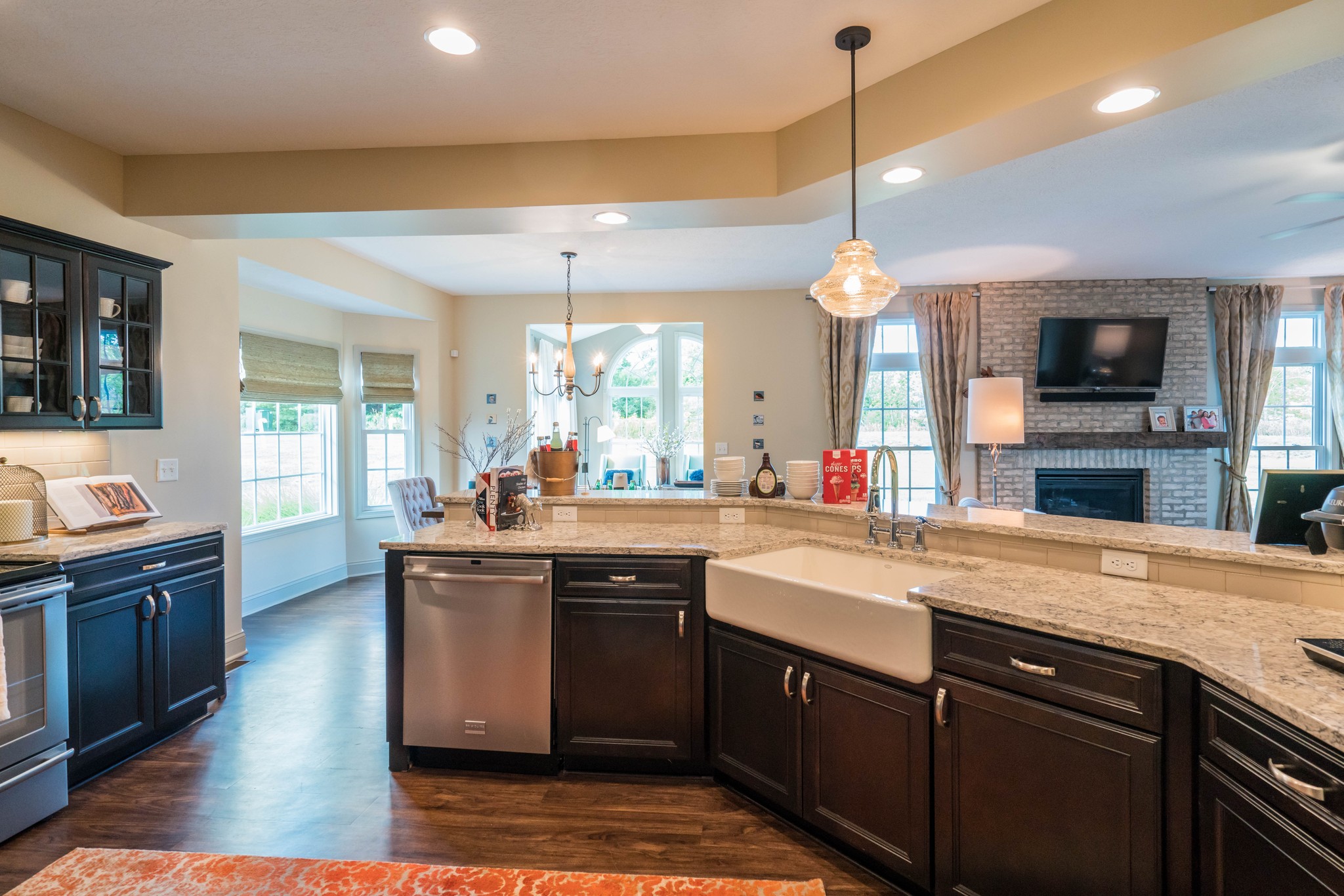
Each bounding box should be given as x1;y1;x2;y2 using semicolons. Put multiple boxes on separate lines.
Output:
687;469;703;480
602;468;640;486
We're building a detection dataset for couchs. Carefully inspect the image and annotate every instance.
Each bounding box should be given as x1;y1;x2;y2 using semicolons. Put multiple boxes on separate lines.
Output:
682;455;703;481
599;452;647;488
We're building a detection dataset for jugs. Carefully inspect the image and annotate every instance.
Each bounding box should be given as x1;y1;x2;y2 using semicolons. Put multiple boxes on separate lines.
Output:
612;473;628;490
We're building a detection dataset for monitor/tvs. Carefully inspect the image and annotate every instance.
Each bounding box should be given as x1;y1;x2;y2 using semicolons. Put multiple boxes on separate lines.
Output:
1034;316;1168;390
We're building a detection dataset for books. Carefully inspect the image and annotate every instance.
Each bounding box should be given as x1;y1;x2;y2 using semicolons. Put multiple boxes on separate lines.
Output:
476;465;527;531
32;474;163;530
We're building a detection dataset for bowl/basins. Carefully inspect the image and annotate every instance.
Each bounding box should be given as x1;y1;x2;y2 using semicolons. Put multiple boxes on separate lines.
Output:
714;466;745;471
714;456;745;464
714;462;745;468
786;460;820;499
101;343;124;374
2;335;43;375
714;470;746;481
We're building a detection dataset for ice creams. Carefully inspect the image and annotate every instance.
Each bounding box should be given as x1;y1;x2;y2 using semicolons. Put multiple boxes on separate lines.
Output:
830;473;844;501
851;472;860;501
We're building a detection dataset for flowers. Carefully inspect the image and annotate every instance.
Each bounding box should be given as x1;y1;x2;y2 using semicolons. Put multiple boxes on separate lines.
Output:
637;421;696;458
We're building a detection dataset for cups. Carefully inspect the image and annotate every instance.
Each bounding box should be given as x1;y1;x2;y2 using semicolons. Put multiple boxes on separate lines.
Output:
587;485;663;490
1;279;38;304
3;396;42;413
100;297;121;318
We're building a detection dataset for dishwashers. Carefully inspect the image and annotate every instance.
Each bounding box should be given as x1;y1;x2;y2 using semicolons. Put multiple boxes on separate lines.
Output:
402;553;555;755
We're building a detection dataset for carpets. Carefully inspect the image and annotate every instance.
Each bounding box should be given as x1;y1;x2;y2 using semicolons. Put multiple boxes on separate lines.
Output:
0;846;829;896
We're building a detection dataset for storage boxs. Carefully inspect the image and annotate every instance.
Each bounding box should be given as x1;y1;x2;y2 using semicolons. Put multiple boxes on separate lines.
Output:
823;449;868;504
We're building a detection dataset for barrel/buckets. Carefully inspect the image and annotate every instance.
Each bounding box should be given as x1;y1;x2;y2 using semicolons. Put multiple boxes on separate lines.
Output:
531;451;583;495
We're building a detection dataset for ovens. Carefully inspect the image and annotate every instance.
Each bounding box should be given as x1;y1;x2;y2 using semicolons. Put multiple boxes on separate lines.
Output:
0;574;74;844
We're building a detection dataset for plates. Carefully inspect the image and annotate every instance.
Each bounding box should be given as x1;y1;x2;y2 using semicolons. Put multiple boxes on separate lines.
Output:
710;479;749;497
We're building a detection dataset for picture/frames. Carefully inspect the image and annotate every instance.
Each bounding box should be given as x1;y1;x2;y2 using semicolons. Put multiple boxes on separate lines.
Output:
1148;406;1177;432
1183;405;1224;432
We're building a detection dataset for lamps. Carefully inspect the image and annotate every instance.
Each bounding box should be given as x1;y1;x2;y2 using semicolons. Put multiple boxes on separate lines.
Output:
809;25;901;319
581;416;617;489
967;377;1025;505
528;252;662;401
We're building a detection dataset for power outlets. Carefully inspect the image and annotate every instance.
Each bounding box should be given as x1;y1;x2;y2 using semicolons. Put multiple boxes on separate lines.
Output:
719;507;745;524
1101;548;1148;580
552;506;577;522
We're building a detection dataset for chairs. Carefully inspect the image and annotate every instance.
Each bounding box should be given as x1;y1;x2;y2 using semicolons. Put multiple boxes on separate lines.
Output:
387;475;444;535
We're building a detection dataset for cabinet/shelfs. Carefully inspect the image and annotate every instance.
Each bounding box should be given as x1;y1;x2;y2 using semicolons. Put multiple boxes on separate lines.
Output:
931;611;1199;895
0;215;176;431
551;556;714;784
1196;678;1344;896
64;533;227;789
706;622;931;896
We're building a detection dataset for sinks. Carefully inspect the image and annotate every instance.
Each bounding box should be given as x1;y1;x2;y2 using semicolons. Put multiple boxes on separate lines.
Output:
703;544;935;685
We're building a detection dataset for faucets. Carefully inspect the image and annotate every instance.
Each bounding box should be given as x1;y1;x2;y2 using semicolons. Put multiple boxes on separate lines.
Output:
869;445;917;549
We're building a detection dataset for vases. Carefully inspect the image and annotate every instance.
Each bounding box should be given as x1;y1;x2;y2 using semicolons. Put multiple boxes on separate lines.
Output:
656;458;671;486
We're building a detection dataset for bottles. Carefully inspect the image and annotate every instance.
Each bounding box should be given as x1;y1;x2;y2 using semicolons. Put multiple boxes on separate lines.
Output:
748;475;757;497
608;480;612;490
755;453;778;498
595;480;601;490
537;422;578;452
629;480;634;490
776;475;786;496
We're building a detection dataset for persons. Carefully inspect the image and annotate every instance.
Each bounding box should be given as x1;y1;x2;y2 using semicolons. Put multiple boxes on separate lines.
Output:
1157;416;1168;426
1187;409;1218;429
